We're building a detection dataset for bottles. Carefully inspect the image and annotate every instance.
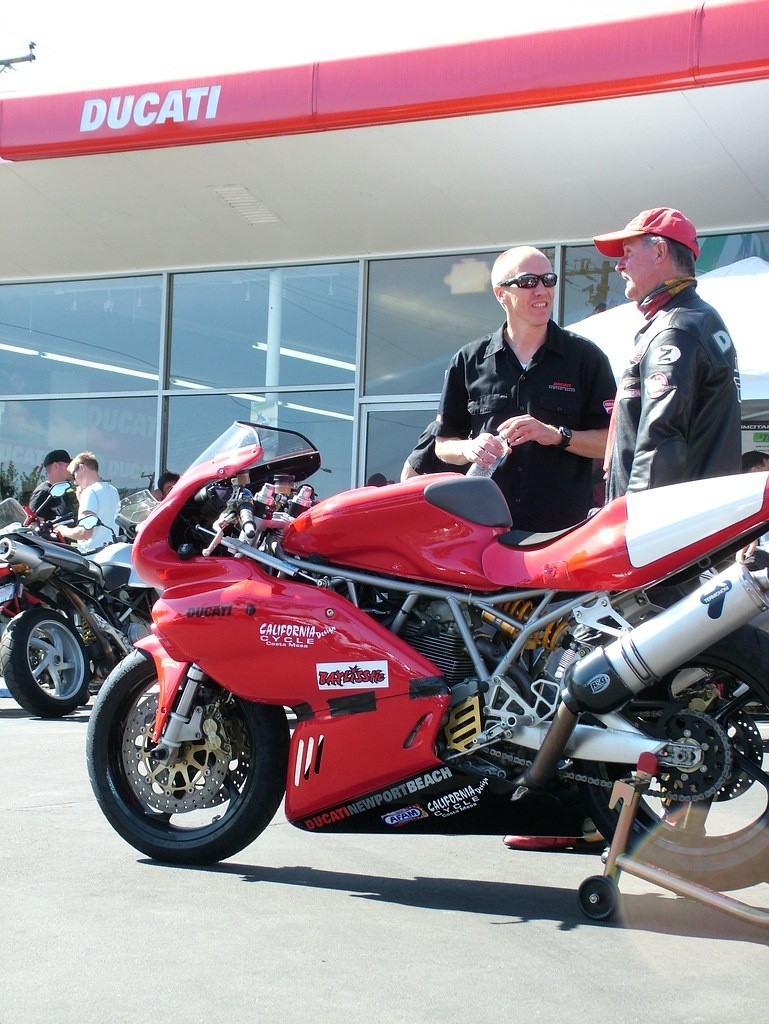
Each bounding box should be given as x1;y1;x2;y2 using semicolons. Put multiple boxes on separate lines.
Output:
464;428;513;478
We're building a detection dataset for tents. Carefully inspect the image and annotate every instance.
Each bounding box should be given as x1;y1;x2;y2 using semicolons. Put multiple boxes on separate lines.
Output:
562;255;769;401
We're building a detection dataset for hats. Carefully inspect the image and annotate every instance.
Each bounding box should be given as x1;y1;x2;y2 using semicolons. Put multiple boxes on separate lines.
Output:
594;207;699;260
44;450;74;467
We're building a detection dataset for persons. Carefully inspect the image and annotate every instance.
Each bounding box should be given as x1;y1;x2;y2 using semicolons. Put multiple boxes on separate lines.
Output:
400;246;617;533
503;206;769;854
28;449;120;553
158;472;181;501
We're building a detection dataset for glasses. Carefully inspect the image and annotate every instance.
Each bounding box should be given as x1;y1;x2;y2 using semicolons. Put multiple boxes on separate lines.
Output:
496;273;558;291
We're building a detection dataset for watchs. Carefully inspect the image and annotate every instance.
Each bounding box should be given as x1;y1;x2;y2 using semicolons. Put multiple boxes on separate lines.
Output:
553;425;572;453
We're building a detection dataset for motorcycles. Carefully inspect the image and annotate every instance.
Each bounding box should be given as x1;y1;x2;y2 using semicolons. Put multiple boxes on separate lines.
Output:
0;489;164;718
82;419;769;931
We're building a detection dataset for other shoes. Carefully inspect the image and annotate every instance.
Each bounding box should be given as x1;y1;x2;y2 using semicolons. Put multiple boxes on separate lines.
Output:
502;831;607;856
